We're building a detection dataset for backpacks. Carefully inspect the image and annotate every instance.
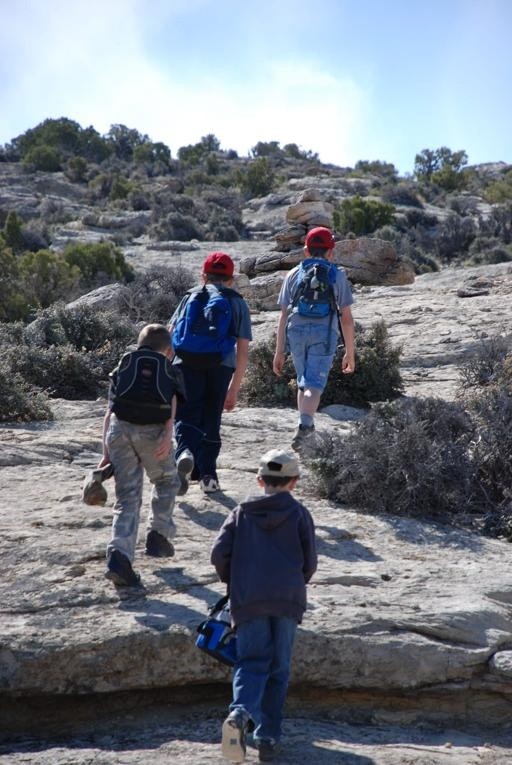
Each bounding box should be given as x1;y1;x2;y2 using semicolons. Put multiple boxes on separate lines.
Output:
111;347;175;426
175;282;240;357
294;261;334;317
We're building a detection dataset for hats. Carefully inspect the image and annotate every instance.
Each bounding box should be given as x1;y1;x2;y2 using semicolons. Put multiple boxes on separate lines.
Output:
304;227;335;247
201;252;235;276
257;448;300;482
80;463;112;508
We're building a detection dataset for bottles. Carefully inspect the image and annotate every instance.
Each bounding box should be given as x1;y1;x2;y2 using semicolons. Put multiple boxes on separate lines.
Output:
216;605;231;624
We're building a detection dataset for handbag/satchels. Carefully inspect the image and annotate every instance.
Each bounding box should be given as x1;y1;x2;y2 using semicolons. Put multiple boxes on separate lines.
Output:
197;597;238;669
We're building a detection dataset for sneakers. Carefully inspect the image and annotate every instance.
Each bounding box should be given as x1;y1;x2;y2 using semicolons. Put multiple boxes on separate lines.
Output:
220;710;249;764
257;741;284;764
201;477;220;493
173;450;194;497
294;424;316;445
147;531;169;550
103;551;140;589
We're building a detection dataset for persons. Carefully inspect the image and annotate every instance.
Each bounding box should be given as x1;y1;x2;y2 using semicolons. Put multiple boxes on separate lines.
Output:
195;449;317;764
272;226;355;451
84;323;183;586
165;251;253;493
